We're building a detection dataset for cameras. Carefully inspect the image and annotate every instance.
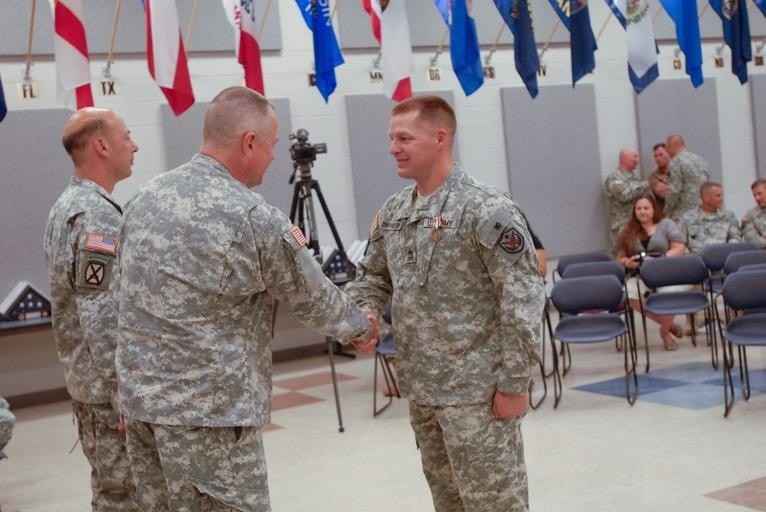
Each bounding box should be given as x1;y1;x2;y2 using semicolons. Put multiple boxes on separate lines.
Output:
288;128;328;165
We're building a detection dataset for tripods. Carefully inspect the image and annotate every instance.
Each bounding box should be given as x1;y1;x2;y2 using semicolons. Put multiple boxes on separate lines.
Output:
272;165;401;432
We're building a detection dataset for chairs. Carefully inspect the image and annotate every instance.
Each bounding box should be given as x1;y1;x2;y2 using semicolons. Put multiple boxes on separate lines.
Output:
529;283;558;410
545;273;636;408
703;242;766;416
562;261;636;376
551;255;627;354
636;255;718;373
374;302;400;415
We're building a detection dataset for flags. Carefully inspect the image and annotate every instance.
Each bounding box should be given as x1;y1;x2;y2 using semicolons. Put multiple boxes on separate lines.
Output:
140;0;195;116
362;0;414;102
433;0;485;96
604;0;765;95
295;0;345;103
549;0;599;89
223;0;264;96
50;0;95;109
493;0;543;100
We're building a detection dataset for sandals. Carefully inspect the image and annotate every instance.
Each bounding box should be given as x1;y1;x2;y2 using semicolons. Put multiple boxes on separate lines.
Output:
665;339;676;350
672;323;682;337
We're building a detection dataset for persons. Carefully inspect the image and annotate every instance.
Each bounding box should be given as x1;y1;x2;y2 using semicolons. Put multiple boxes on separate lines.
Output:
604;146;655;247
647;142;671;219
614;193;688;350
522;212;547;277
677;182;744;336
663;133;711;222
113;84;375;511
42;107;141;511
739;178;765;244
344;94;547;510
